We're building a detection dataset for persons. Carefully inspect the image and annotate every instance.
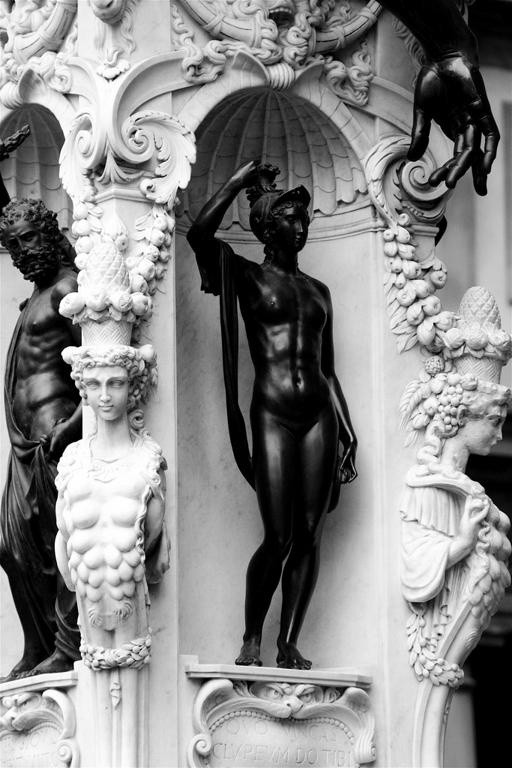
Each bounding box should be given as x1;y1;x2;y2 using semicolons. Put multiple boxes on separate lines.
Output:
1;124;80;681
184;159;359;671
374;0;501;196
399;370;511;691
57;343;169;672
